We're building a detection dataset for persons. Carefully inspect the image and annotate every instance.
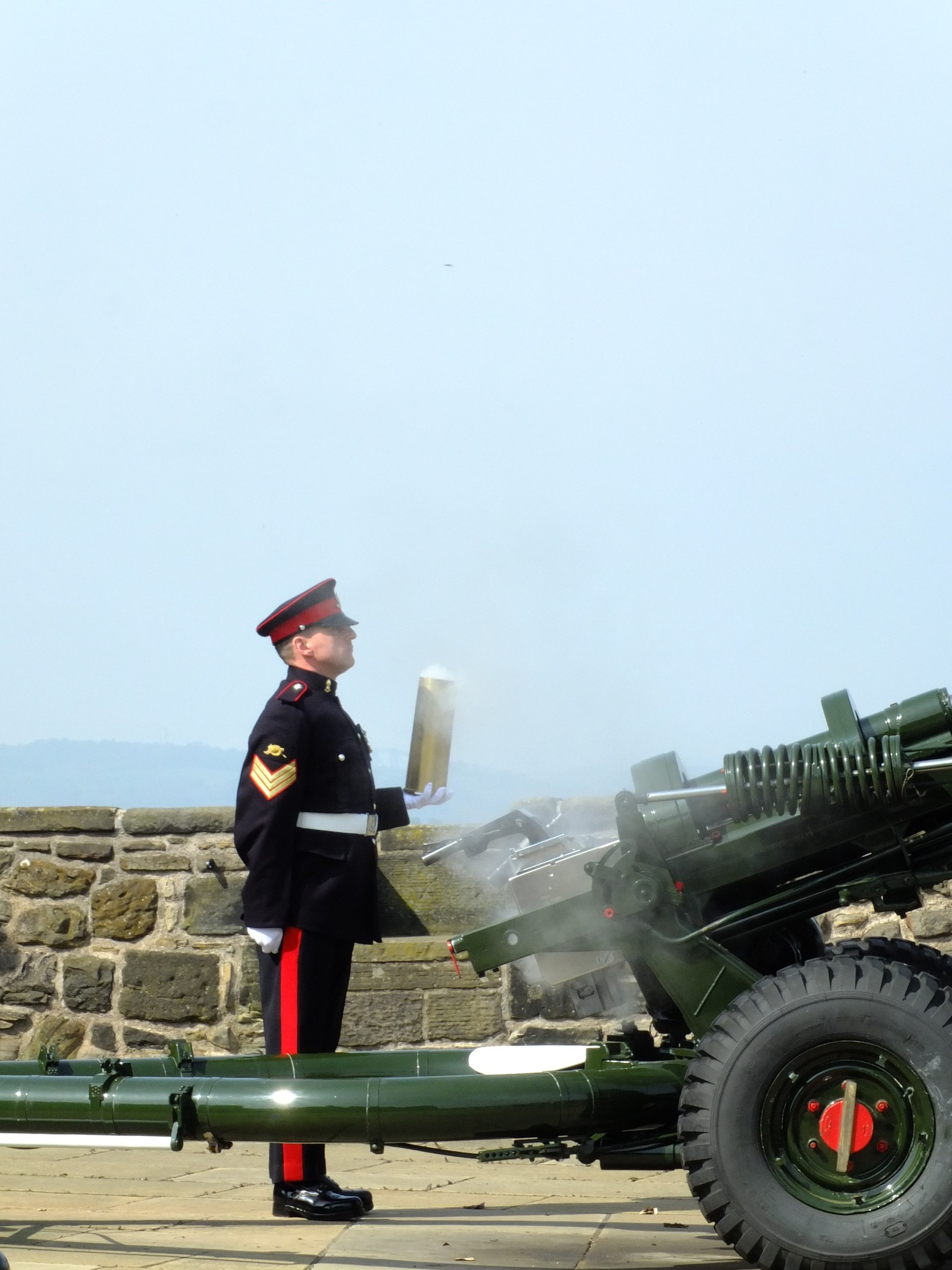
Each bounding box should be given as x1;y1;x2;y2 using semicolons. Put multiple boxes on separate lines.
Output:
233;579;431;1218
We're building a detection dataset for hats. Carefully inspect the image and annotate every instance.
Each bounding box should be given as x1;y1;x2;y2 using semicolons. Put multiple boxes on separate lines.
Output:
255;577;358;647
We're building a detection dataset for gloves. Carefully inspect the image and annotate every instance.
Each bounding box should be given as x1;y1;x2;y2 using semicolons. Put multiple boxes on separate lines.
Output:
404;782;454;810
247;927;283;954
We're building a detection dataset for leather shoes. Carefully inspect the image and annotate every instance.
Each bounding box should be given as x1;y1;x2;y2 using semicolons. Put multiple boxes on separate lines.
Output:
320;1174;373;1212
273;1176;364;1219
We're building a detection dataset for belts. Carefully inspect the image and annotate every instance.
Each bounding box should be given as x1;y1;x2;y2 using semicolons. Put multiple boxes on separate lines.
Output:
295;811;378;836
